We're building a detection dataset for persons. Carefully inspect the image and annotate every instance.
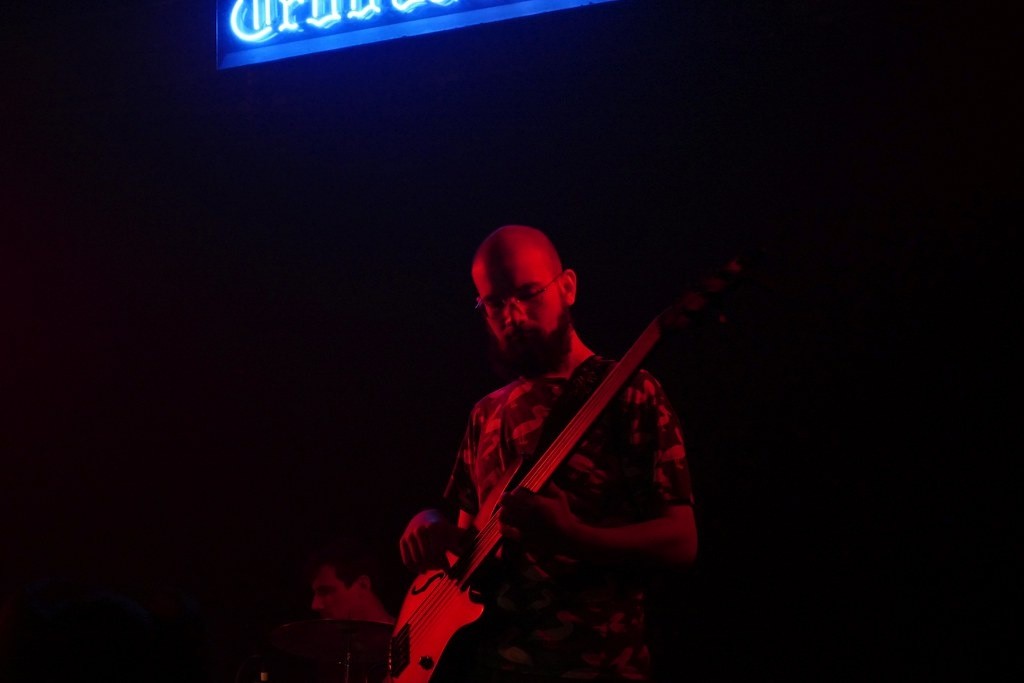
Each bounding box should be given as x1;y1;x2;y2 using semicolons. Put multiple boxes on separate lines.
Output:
394;222;700;683
306;522;400;633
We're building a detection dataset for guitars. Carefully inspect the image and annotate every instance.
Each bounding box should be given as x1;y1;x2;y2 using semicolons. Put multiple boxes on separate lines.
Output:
383;258;743;683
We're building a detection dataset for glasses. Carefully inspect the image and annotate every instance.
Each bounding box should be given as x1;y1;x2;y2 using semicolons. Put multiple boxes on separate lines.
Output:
474;273;561;316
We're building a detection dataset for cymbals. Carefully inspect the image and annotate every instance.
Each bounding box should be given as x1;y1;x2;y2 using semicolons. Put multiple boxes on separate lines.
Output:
271;617;394;665
